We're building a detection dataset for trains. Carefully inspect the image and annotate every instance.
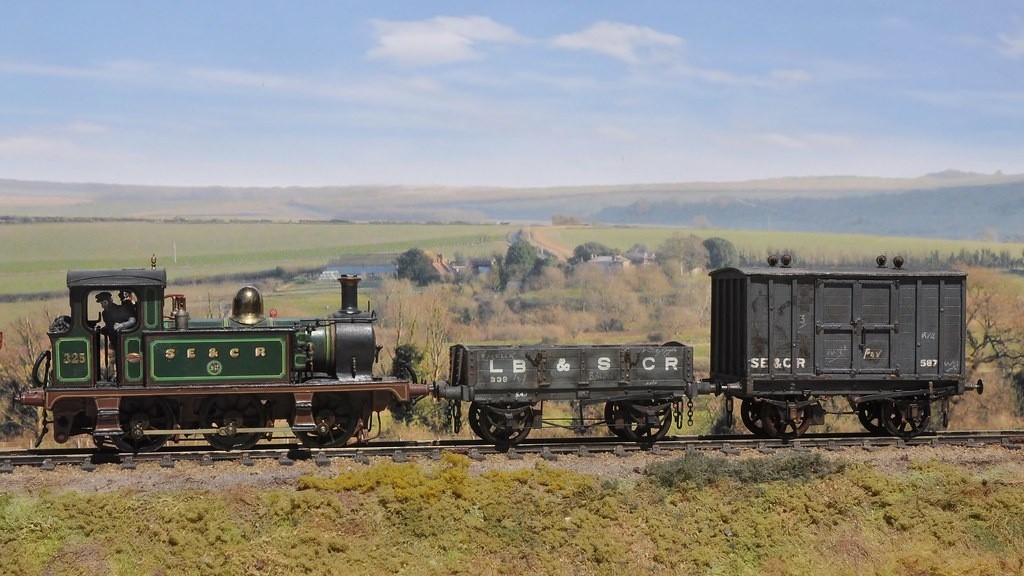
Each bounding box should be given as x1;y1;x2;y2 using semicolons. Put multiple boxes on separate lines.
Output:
13;256;985;440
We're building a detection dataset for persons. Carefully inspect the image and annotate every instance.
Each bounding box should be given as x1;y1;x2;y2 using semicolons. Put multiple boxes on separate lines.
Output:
94;292;136;347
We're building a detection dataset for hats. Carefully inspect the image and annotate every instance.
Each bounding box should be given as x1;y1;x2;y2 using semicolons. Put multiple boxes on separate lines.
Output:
95;292;112;302
